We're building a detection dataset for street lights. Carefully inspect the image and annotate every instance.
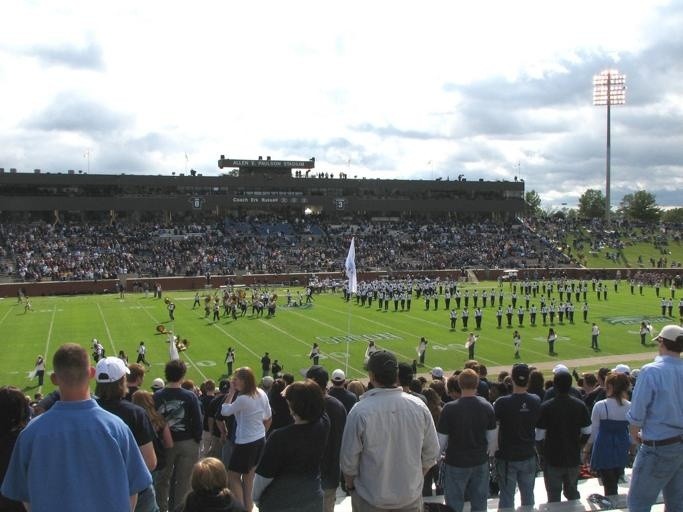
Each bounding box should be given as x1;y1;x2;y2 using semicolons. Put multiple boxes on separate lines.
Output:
591;66;627;226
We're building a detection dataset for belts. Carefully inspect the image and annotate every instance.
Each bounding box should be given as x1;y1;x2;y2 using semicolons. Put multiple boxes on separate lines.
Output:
641;436;683;446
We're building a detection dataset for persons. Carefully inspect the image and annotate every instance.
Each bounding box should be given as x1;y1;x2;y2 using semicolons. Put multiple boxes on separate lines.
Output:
0;170;683;512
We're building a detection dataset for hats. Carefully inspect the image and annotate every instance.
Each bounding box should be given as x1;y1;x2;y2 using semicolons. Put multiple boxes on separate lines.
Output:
611;364;630;373
651;325;683;342
429;367;444;378
512;363;528;381
96;356;131;383
332;369;345;383
366;351;397;371
553;364;568;373
